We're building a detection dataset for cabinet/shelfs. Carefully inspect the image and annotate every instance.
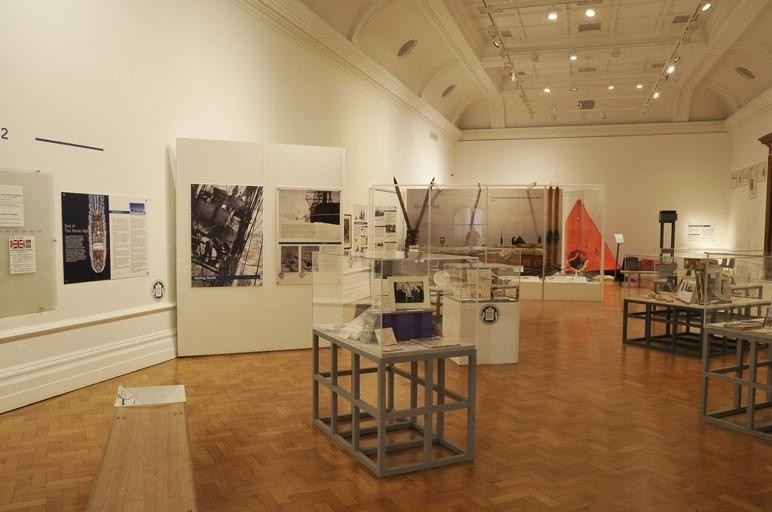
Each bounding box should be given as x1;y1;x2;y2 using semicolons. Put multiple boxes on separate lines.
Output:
442;262;520;368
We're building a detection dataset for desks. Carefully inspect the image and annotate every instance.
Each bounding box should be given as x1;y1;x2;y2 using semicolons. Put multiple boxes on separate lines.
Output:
622;297;771;359
310;326;476;480
701;324;772;442
674;253;733;259
622;269;660;285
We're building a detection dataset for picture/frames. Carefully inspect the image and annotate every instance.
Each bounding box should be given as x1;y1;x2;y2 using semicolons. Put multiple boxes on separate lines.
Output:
390;274;430;309
